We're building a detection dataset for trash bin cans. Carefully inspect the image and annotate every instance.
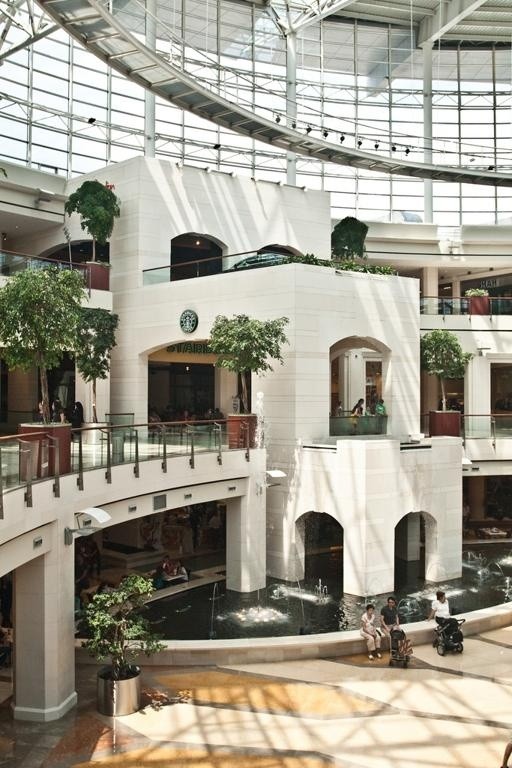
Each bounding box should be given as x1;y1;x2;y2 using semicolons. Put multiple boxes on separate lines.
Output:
111;431;125;465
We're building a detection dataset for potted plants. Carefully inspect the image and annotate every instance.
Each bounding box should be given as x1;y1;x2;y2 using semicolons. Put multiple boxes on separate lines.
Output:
464;288;490;315
79;573;169;718
208;313;291;449
421;329;475;436
0;261;120;484
64;180;120;290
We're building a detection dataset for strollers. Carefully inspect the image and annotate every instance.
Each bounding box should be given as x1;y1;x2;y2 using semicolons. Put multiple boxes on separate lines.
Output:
432;619;465;656
389;628;413;668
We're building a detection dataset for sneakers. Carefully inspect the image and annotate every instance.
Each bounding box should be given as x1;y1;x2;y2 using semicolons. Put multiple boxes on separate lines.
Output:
368;655;374;659
377;653;382;658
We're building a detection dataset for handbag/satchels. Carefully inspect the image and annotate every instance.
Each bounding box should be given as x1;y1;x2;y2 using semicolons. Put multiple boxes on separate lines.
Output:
397;639;413;657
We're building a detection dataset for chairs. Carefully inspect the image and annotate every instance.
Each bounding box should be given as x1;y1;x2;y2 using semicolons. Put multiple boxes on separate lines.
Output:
84;591;93;603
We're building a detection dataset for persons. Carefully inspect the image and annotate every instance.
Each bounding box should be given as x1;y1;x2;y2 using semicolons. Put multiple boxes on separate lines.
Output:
337;397;386;433
161;401;228;434
463;500;471;533
360;605;381;659
208;509;223;553
380;597;399;653
501;739;512;768
153;555;188;590
39;397;82;443
425;591;451;645
